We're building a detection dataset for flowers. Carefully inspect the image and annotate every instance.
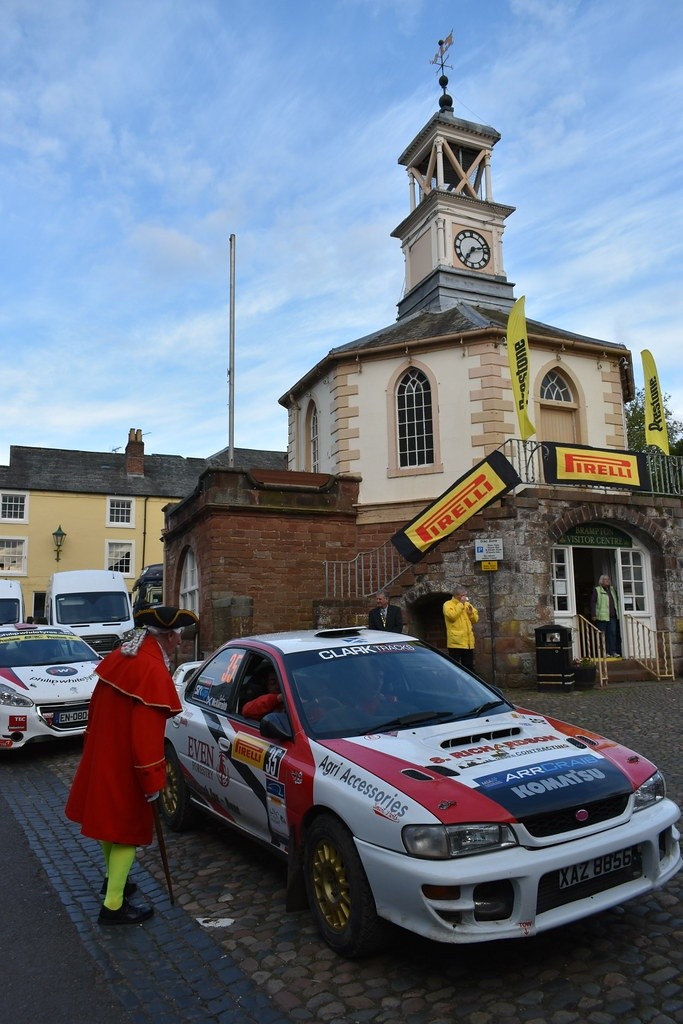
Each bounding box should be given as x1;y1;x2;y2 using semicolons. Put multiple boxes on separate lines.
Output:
573;658;595;667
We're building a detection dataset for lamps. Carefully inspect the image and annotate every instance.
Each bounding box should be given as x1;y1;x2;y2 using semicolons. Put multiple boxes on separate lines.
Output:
597;351;607;369
556;343;566;361
495;337;507;348
52;524;67;562
613;357;628;369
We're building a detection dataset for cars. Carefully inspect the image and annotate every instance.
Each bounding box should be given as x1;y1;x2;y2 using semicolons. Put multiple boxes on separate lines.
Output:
0;624;105;756
151;626;683;955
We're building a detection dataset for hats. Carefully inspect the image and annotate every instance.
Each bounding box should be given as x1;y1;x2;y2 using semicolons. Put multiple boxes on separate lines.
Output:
135;607;198;629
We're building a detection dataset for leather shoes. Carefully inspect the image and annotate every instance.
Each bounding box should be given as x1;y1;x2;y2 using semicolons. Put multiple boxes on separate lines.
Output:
98;897;153;924
101;876;137;894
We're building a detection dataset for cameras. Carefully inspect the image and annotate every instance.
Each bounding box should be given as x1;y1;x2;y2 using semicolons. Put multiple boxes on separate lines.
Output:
465;597;471;601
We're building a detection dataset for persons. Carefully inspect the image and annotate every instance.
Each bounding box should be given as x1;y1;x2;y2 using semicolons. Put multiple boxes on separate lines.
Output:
65;605;198;925
356;665;400;715
592;574;620;658
241;666;326;728
442;586;478;674
369;588;403;634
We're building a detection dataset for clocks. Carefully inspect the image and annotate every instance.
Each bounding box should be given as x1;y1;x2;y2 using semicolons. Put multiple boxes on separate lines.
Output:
454;230;490;270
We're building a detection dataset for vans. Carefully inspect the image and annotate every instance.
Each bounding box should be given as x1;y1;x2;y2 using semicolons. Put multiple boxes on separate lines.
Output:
38;563;163;658
0;580;34;629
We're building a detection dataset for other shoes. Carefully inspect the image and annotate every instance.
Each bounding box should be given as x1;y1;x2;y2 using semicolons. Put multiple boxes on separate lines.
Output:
613;653;620;658
606;653;610;658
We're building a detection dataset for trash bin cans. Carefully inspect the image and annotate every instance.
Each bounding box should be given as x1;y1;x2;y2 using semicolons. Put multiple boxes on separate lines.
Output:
534;625;575;693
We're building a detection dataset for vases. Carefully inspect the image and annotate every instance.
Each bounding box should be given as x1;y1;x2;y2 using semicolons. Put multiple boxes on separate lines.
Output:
574;667;597;691
